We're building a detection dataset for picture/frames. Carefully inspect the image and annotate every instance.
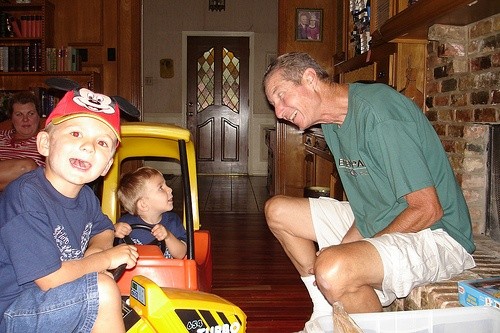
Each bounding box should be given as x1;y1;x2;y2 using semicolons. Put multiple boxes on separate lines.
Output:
294;8;323;43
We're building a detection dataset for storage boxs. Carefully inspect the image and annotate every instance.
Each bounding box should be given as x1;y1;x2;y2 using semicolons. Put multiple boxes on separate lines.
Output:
457;275;500;312
304;306;500;333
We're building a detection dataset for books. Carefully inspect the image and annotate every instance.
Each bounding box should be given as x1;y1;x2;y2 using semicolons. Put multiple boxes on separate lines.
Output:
45;47;82;72
0;41;41;71
34;90;59;118
0;13;43;39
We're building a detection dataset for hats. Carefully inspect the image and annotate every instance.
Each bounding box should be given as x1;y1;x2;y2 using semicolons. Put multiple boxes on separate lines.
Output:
44;78;140;147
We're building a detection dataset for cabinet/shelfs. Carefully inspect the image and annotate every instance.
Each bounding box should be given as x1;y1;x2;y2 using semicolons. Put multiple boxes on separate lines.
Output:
1;0;142;144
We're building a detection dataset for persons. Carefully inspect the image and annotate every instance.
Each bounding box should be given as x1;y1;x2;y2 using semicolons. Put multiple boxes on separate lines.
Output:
262;52;478;333
0;88;139;333
307;16;319;40
111;168;187;261
0;92;48;191
298;15;308;39
